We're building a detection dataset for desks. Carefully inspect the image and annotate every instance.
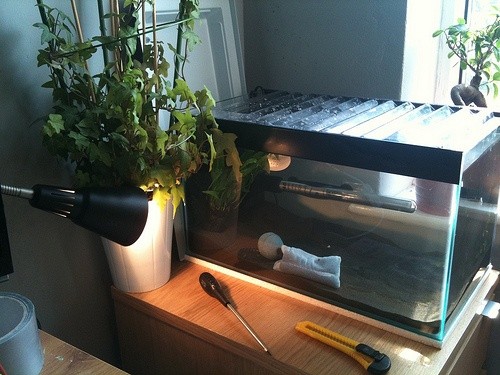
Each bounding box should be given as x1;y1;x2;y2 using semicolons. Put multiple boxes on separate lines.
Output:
111;257;500;375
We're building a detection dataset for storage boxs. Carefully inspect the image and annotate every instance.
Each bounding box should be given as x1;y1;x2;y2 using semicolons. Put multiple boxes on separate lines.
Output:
180;86;500;349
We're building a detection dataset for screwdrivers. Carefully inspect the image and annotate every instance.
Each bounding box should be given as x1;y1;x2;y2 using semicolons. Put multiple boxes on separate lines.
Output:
198;272;269;354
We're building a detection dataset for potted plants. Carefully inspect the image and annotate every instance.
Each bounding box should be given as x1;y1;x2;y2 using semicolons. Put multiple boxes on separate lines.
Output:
32;0;268;295
